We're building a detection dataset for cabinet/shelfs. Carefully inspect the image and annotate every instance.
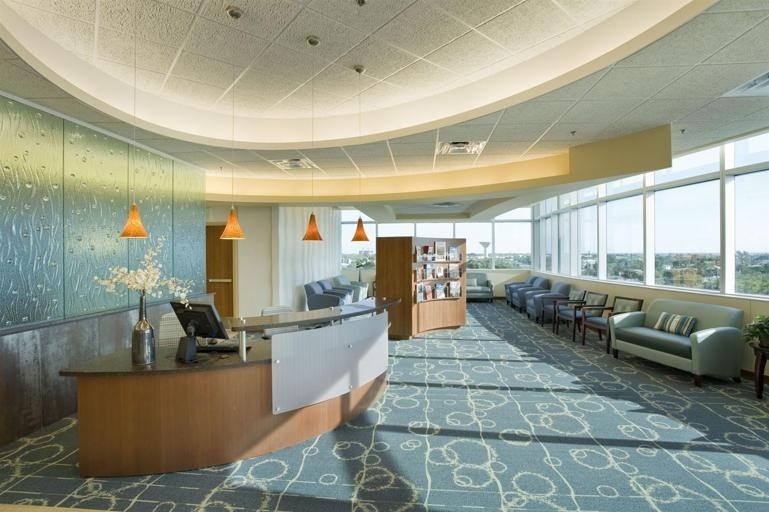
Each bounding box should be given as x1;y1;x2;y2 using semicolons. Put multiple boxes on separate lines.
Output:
375;235;467;339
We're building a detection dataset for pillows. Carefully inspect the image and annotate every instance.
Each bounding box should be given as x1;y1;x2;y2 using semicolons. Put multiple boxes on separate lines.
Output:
654;311;697;337
467;278;477;286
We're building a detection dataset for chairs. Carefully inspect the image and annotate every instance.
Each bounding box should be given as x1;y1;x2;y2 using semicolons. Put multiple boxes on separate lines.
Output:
582;296;644;353
504;275;570;324
542;287;586;334
466;272;495;303
556;291;607;342
304;274;369;310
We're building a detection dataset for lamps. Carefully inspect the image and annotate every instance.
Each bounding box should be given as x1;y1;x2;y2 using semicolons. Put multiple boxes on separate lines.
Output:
302;35;323;241
351;63;369;241
118;0;150;238
219;4;245;240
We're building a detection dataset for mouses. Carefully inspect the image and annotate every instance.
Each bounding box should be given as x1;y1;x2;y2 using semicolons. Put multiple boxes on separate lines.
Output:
207;338;217;344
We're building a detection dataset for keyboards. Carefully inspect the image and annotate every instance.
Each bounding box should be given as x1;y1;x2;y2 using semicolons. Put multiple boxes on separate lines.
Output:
194;343;252;352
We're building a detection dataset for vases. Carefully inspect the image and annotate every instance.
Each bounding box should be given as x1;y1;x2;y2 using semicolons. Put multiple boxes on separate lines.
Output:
131;295;155;365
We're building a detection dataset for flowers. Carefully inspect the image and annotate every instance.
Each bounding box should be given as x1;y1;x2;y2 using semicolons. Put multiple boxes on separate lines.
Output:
94;237;200;310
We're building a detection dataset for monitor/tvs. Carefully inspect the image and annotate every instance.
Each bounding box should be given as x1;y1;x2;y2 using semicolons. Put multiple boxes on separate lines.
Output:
170;301;229;364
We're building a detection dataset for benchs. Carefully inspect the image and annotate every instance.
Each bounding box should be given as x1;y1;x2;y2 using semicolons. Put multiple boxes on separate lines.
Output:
608;298;744;387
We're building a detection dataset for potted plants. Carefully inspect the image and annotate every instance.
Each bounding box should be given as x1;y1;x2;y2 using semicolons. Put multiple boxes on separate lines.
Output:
742;313;769;348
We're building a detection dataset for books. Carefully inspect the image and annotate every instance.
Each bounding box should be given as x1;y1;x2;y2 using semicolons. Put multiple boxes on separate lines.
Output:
415;241;460;301
